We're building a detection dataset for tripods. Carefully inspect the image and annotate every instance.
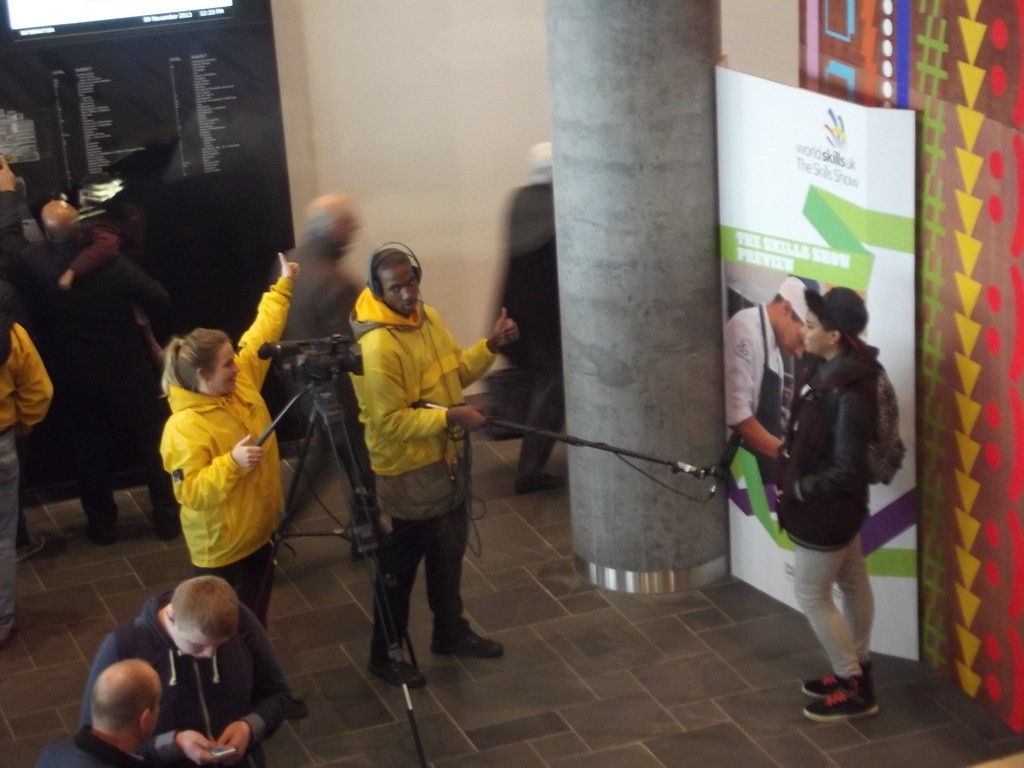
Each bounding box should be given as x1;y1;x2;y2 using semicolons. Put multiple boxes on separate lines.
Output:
247;365;429;768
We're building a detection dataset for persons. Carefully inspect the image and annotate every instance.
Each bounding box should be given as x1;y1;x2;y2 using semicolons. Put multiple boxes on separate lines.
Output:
775;285;908;724
485;143;566;493
0;154;184;546
276;194;389;558
724;277;809;482
347;251;522;684
35;660;163;768
0;278;53;645
81;575;290;768
160;251;315;719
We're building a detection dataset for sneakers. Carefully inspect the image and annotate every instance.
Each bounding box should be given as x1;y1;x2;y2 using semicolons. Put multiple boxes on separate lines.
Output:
803;660;872;698
432;632;503;659
367;656;426;688
803;672;878;720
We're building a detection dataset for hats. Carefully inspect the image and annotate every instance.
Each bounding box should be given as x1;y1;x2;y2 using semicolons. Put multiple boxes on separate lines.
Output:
804;286;867;349
778;277;809;324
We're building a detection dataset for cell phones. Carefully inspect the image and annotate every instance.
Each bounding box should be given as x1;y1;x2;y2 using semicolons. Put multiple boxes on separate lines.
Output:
210;744;237;758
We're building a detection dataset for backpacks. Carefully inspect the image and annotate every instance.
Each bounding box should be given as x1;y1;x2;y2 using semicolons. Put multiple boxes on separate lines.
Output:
867;366;906;485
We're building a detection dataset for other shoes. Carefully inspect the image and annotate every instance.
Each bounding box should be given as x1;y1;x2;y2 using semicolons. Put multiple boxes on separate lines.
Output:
16;534;45;562
515;473;565;494
155;527;180;540
284;698;310;720
86;526;115;544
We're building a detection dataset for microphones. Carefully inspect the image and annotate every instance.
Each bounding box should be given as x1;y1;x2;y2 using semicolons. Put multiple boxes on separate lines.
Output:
710;430;742;496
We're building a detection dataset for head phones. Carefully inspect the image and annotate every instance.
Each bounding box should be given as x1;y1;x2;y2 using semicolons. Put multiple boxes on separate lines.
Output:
367;240;422;296
